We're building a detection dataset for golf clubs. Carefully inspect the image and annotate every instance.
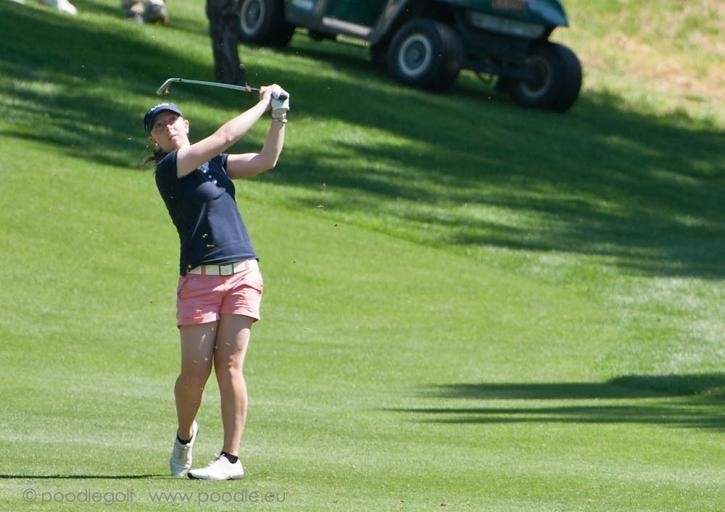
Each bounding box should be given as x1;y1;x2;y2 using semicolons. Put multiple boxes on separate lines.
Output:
157;78;287;100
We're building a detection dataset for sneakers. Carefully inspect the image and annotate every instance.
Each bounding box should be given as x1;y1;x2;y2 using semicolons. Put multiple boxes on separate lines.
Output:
170;420;199;477
188;453;244;480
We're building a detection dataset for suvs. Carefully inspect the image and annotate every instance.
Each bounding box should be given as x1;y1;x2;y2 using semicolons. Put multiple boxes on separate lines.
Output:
239;0;581;112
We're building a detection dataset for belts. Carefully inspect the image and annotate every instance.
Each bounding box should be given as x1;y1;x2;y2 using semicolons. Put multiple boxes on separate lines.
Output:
186;259;257;276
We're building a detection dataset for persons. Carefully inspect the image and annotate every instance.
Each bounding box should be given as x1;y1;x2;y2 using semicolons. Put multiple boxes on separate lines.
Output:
144;84;290;480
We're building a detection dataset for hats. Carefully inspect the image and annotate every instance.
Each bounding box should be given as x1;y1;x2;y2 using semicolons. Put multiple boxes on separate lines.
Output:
143;102;182;136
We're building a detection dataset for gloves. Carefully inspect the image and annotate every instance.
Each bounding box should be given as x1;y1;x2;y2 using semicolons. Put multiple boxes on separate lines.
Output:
266;84;289;111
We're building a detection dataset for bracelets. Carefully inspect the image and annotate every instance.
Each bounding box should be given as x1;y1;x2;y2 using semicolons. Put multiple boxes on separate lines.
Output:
272;113;287;123
